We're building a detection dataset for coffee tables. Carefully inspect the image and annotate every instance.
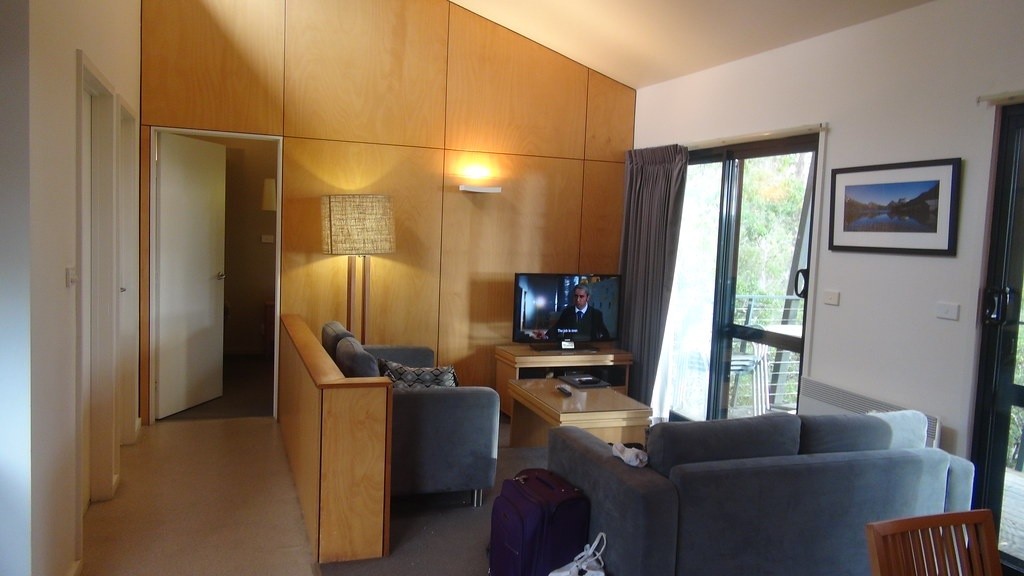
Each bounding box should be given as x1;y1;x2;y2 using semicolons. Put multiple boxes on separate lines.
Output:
506;378;653;448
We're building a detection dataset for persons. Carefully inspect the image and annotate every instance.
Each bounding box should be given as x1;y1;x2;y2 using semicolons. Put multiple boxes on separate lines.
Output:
533;283;610;341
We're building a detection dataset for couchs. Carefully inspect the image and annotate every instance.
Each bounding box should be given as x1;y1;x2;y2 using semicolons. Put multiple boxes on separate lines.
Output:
549;410;975;576
321;320;500;507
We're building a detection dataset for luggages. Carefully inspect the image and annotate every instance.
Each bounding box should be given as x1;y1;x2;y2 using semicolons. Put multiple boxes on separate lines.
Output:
486;468;591;576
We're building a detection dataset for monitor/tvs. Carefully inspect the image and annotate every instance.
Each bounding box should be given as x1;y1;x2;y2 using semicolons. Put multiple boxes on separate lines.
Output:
512;273;622;352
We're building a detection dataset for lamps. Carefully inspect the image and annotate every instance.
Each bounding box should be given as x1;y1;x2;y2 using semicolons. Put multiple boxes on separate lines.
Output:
459;184;501;194
321;194;396;345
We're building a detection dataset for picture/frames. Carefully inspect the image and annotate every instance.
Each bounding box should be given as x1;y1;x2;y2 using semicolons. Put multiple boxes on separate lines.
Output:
828;158;962;256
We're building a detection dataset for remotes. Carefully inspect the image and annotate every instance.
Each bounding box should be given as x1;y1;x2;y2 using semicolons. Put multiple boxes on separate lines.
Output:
557;387;572;397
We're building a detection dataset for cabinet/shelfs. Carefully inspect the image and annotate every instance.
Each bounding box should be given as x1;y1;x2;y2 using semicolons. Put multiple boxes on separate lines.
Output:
493;345;633;418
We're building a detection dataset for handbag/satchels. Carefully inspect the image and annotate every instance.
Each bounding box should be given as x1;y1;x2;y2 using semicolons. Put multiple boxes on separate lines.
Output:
547;532;607;576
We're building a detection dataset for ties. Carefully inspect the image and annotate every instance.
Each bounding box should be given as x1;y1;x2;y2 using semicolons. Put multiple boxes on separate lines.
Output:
577;311;583;324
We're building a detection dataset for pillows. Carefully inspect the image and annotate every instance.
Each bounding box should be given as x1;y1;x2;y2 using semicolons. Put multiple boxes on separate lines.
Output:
379;358;459;387
383;370;447;388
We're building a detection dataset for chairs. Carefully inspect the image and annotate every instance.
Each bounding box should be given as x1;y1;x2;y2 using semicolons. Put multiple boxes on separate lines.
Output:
866;508;1003;576
725;324;770;418
